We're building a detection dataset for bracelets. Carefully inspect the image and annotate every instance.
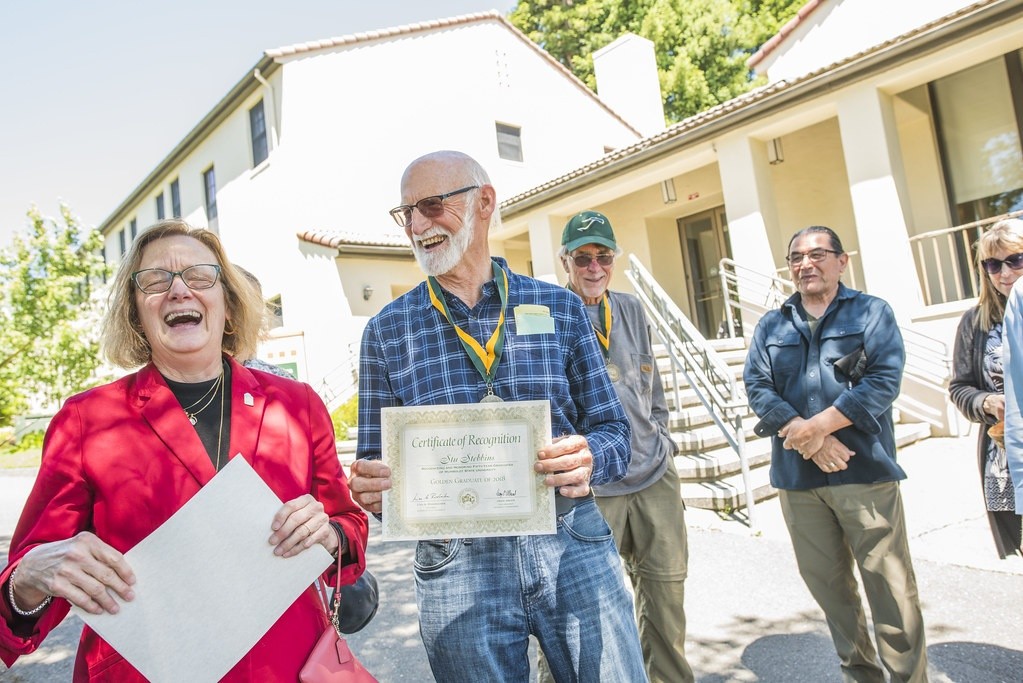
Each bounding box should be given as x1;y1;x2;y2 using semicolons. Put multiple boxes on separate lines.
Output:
329;519;347;559
8;570;52;616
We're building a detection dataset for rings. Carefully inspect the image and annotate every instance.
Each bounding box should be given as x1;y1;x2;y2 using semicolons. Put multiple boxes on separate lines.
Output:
303;523;311;536
828;462;834;468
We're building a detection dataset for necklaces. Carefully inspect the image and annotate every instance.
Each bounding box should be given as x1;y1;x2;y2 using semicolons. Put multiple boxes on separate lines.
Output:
183;372;224;427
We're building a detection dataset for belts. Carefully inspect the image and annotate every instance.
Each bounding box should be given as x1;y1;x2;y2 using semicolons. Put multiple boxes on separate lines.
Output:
555;491;594;516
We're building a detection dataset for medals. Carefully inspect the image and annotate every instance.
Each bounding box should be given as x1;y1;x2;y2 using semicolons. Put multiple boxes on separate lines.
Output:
480;391;504;404
605;363;621;383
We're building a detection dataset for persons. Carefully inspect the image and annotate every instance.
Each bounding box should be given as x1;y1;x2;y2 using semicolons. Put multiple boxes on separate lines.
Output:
538;211;697;683
743;226;929;683
225;262;297;382
343;150;647;683
0;222;371;683
946;217;1023;561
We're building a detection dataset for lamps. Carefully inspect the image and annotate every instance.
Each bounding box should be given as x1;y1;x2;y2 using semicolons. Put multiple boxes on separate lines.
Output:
364;284;373;300
660;178;677;205
766;136;784;165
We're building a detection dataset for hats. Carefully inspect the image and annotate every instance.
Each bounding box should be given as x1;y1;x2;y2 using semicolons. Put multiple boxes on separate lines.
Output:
561;211;616;251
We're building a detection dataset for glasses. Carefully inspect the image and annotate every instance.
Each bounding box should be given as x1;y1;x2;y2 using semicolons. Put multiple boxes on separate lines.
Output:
131;264;220;294
389;186;482;227
568;254;614;268
786;248;842;263
980;252;1023;274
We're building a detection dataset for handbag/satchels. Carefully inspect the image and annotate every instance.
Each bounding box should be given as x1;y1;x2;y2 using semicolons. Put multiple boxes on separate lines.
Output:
299;523;380;683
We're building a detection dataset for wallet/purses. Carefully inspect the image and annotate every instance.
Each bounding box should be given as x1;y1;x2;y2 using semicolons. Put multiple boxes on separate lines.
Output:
834;346;868;383
